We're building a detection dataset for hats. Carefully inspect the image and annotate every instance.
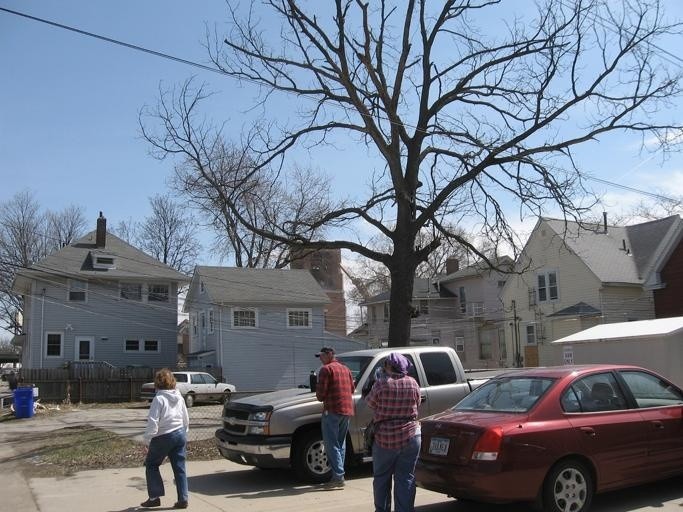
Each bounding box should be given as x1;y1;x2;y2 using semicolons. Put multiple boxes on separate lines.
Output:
314;346;336;357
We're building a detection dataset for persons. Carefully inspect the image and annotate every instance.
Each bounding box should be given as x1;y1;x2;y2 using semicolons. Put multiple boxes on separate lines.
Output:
314;345;356;489
140;367;189;509
363;350;423;512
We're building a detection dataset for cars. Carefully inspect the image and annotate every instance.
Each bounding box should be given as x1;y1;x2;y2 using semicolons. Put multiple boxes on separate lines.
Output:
415;364;682;512
140;371;236;408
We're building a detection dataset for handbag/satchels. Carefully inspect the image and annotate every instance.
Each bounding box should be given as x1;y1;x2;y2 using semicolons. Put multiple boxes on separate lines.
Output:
363;420;375;450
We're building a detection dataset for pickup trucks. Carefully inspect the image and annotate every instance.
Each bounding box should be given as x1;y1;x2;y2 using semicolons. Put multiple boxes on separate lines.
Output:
215;346;495;485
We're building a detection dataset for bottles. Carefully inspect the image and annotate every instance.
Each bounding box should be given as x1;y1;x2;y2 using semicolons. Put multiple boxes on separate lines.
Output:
310;371;318;393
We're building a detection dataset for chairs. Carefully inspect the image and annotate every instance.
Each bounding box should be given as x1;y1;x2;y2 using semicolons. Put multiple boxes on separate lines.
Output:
592;384;618;410
569;391;586;411
492;390;580;413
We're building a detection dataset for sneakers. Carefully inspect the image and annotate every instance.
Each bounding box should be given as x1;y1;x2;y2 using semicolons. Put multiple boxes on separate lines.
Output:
173;500;188;508
140;498;161;507
319;475;345;491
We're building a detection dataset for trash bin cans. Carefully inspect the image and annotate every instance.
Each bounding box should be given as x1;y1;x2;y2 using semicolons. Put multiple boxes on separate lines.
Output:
12;386;33;418
